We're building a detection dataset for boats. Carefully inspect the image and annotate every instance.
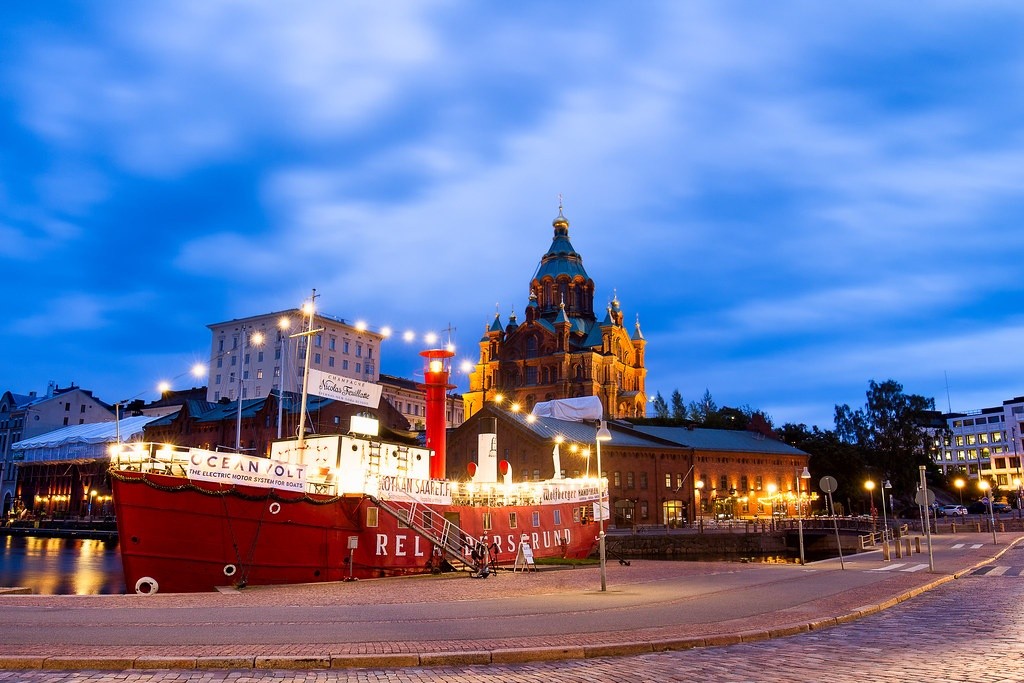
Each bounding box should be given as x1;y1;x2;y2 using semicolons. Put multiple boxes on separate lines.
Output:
106;287;613;594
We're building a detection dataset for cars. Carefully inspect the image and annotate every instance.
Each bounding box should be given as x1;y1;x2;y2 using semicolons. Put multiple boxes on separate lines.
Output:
932;504;969;518
898;505;934;519
988;501;1012;514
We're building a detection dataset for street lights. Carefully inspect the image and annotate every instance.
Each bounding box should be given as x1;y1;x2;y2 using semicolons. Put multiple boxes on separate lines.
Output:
881;480;892;542
795;466;813;565
955;478;966;524
595;420;613;591
980;479;997;545
696;480;705;534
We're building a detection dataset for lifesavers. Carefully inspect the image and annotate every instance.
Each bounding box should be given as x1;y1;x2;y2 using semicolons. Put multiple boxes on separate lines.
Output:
268;502;281;514
223;564;237;576
135;576;159;595
